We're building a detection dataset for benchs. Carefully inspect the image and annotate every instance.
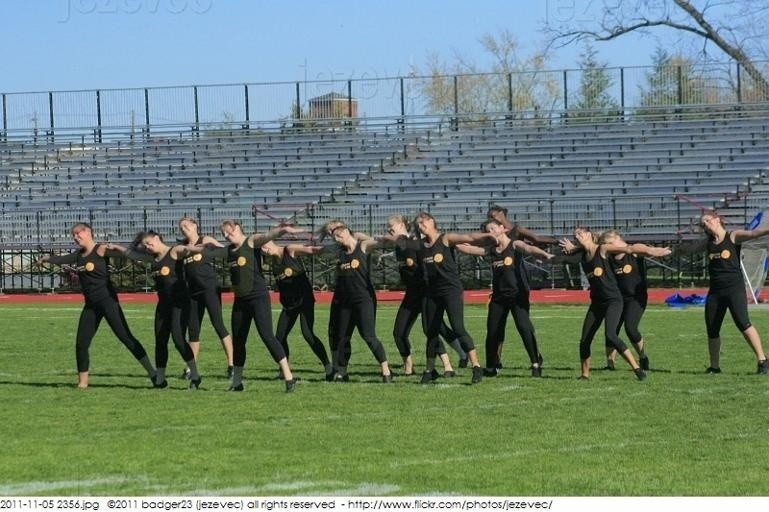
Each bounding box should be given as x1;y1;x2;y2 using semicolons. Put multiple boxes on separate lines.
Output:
2;108;767;253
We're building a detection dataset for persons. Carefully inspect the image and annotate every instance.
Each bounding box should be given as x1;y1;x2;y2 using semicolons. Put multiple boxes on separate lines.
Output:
32;206;769;394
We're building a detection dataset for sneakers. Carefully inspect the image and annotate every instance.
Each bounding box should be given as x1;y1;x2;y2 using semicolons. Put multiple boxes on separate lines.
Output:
277;374;299;394
325;367;351;383
419;369;440;384
443;370;456;379
402;369;417;376
383;370;395;383
604;365;616;370
577;375;588;380
532;354;543;377
704;367;722;375
226;365;244;392
459;353;468;370
182;369;203;392
470;364;502;384
148;374;168;390
635;357;649;382
758;358;769;376
